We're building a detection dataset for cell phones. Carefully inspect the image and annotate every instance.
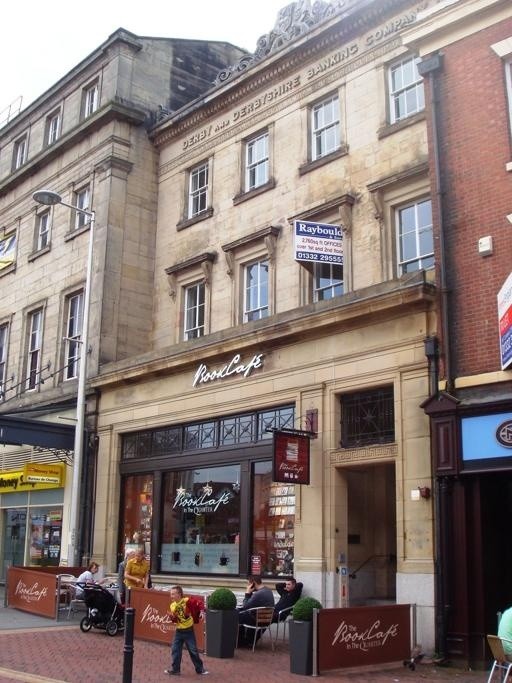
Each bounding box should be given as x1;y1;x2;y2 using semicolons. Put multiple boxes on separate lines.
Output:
251;583;254;589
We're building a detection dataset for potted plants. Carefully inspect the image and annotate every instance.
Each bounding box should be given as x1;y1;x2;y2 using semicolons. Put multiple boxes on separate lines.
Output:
287;596;322;676
204;588;239;659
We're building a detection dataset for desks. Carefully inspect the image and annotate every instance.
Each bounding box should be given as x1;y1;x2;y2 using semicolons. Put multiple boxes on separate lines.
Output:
96;582;128;606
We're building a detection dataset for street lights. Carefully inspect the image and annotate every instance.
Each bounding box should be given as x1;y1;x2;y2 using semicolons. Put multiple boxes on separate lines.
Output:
31;190;96;567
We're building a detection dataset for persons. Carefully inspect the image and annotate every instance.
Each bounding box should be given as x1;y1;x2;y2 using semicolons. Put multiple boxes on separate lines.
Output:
236;575;274;624
166;586;208;675
117;547;150;604
498;607;512;662
76;563;108;599
273;578;303;623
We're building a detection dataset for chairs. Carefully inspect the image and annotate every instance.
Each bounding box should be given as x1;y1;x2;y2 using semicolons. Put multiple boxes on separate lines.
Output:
483;633;512;683
54;573;91;620
235;602;297;654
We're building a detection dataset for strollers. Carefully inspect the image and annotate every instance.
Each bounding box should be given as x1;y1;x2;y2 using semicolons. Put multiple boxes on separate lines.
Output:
76;582;125;637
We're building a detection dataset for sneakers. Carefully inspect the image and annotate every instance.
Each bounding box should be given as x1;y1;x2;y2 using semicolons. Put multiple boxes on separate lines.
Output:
197;670;209;674
164;670;180;675
